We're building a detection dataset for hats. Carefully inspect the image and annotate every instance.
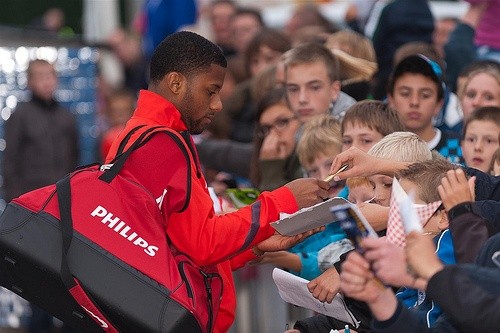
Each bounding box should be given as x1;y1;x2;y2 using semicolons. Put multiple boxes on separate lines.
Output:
388;54;446;100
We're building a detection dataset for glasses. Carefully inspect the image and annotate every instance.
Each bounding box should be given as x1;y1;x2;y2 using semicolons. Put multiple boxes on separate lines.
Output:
256;117;297;139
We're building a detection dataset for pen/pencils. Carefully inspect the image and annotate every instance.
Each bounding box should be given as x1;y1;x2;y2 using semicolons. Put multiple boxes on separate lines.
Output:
285;323;288;331
365;196;376;203
324;165;348;183
345;325;349;333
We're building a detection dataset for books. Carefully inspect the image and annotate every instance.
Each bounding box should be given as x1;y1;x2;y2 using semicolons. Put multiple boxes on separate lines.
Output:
272;267;359;328
330;204;391;289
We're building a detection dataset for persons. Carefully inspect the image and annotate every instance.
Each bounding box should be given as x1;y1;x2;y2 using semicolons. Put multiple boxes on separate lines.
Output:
96;0;500;333
105;31;330;333
0;59;81;333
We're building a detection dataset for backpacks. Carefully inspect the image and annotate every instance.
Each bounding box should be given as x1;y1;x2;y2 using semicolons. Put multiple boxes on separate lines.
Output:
0;123;224;333
